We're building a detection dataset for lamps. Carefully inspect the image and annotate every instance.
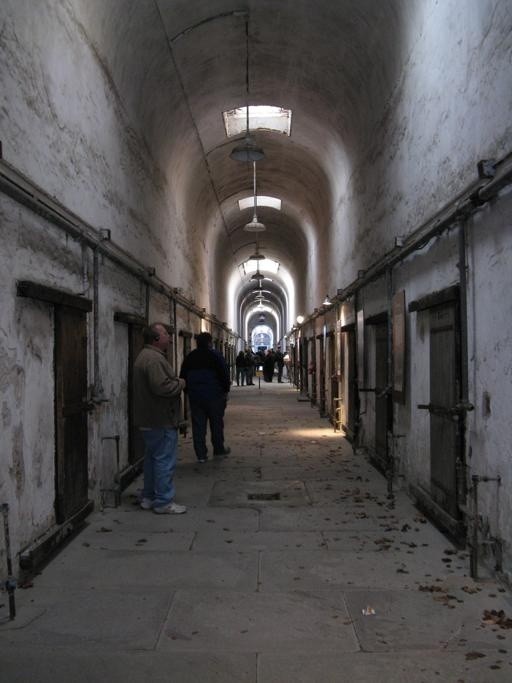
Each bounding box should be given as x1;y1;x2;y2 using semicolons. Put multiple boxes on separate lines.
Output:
227;10;269;324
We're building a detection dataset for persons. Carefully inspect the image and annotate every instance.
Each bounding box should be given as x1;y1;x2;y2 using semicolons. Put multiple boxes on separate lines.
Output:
180;330;232;465
234;342;291;386
134;320;189;514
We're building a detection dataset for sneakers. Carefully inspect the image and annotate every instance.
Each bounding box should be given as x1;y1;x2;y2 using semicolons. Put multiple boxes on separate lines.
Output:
140;497;186;514
195;447;231;463
